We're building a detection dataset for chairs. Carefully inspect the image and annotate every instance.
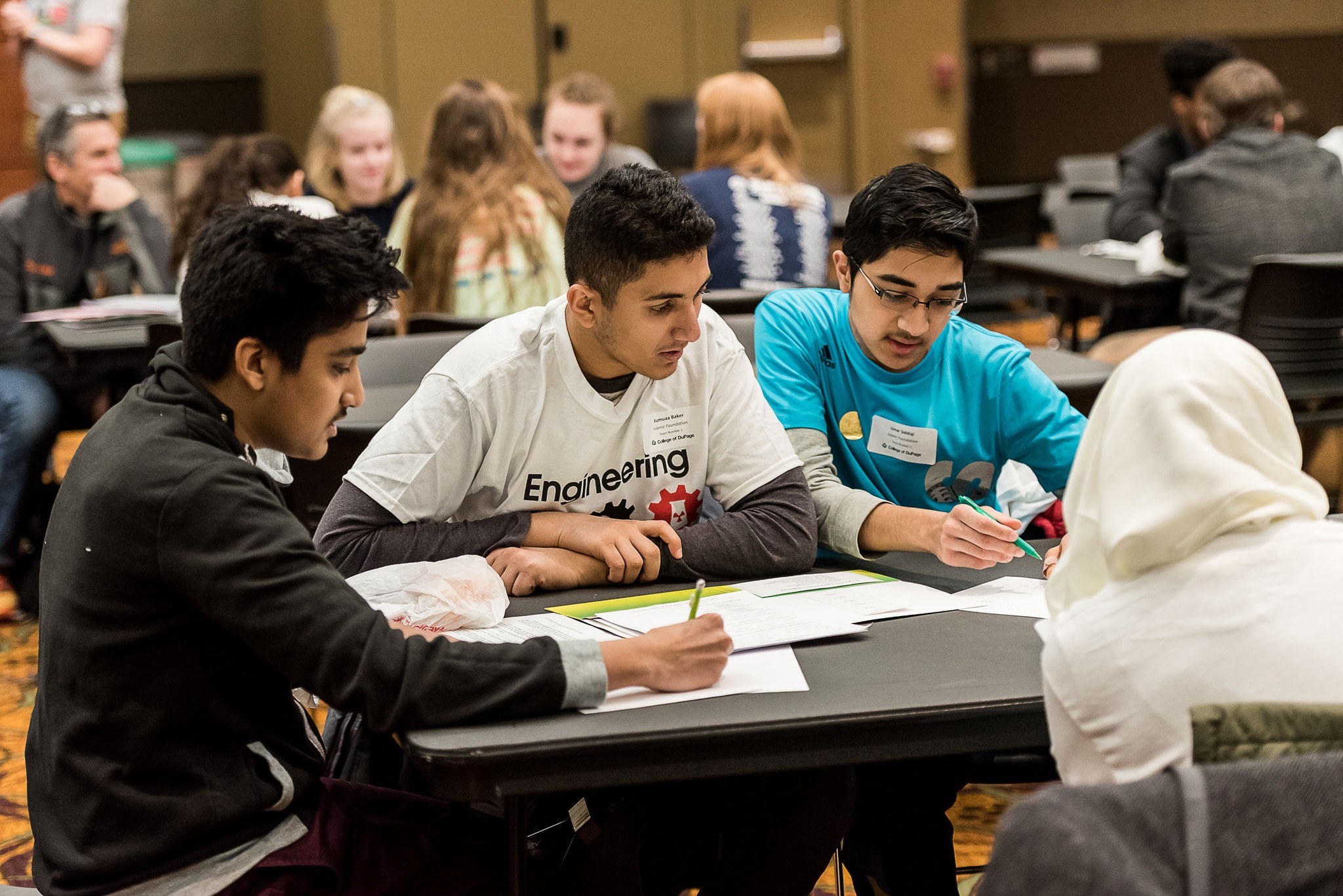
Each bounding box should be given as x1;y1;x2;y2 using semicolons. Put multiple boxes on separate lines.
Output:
146;153;1343;896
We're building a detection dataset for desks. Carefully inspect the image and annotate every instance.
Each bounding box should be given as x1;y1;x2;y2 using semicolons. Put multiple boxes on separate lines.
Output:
38;320;400;428
980;242;1178;351
398;539;1047;795
338;346;1112;417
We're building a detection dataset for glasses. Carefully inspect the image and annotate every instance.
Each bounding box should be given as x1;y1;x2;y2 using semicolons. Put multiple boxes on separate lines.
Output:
848;255;967;316
47;101;109;150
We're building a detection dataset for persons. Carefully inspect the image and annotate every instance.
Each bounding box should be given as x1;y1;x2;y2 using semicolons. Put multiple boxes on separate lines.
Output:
1033;330;1343;788
0;100;183;625
0;0;129;158
310;161;854;896
754;164;1089;896
25;202;731;896
166;73;835;316
1106;39;1343;336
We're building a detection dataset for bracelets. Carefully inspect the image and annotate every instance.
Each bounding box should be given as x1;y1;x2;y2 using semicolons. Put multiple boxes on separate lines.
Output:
21;22;44;48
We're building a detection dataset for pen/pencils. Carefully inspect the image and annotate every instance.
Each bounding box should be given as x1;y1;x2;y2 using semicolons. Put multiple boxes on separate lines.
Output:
688;579;706;619
958;495;1044;561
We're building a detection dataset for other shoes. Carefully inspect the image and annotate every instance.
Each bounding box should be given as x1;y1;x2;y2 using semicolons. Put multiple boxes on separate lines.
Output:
0;592;33;624
